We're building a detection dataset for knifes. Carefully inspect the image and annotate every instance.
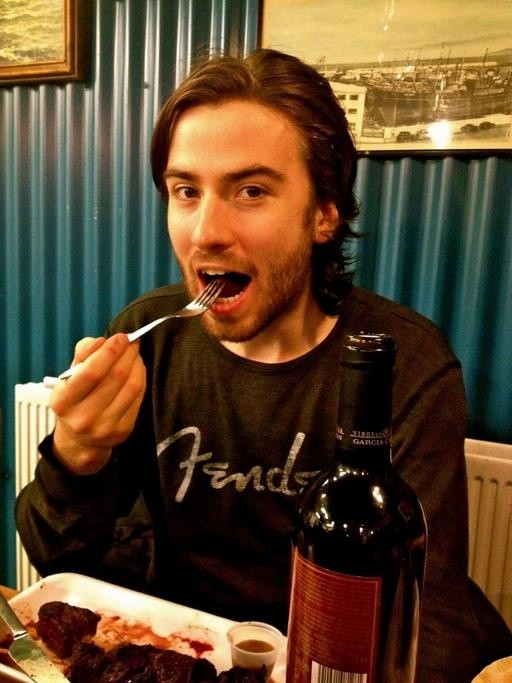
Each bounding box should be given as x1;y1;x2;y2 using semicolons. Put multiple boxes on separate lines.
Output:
0;591;73;683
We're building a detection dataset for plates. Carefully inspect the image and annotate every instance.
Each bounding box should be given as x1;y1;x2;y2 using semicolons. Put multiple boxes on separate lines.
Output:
0;571;288;683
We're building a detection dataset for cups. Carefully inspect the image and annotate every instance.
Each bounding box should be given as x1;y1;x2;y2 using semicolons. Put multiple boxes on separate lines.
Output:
226;620;285;683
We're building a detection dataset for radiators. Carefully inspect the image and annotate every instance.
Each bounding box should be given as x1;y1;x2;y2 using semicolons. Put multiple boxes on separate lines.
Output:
14;381;512;635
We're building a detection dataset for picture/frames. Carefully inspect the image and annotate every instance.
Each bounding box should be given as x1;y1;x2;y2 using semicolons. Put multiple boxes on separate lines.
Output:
254;0;512;157
0;0;88;87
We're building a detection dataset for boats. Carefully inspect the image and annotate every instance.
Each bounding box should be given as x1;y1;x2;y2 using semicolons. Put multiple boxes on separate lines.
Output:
319;42;510;129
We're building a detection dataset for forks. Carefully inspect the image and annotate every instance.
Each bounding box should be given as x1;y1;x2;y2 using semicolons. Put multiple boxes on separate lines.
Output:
58;278;229;382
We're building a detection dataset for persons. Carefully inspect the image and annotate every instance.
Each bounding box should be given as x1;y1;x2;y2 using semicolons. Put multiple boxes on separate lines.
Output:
15;45;510;679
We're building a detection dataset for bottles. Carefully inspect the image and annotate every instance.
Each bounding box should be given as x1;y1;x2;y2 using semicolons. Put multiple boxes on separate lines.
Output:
286;328;430;683
